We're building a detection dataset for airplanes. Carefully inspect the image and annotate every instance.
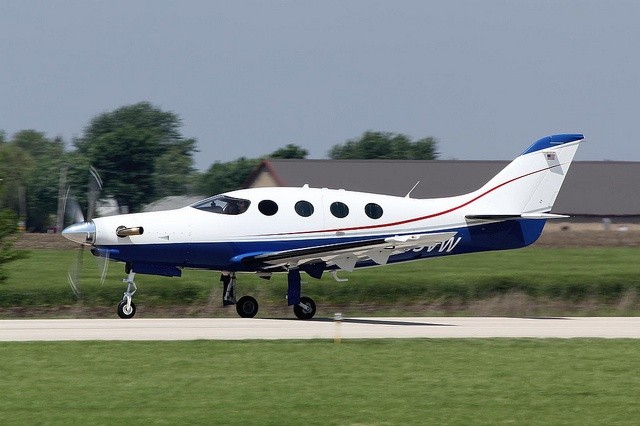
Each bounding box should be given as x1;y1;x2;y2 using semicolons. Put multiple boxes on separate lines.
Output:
57;134;585;320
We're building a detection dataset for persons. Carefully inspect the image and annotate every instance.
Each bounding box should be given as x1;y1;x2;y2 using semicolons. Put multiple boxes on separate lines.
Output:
237;200;248;213
